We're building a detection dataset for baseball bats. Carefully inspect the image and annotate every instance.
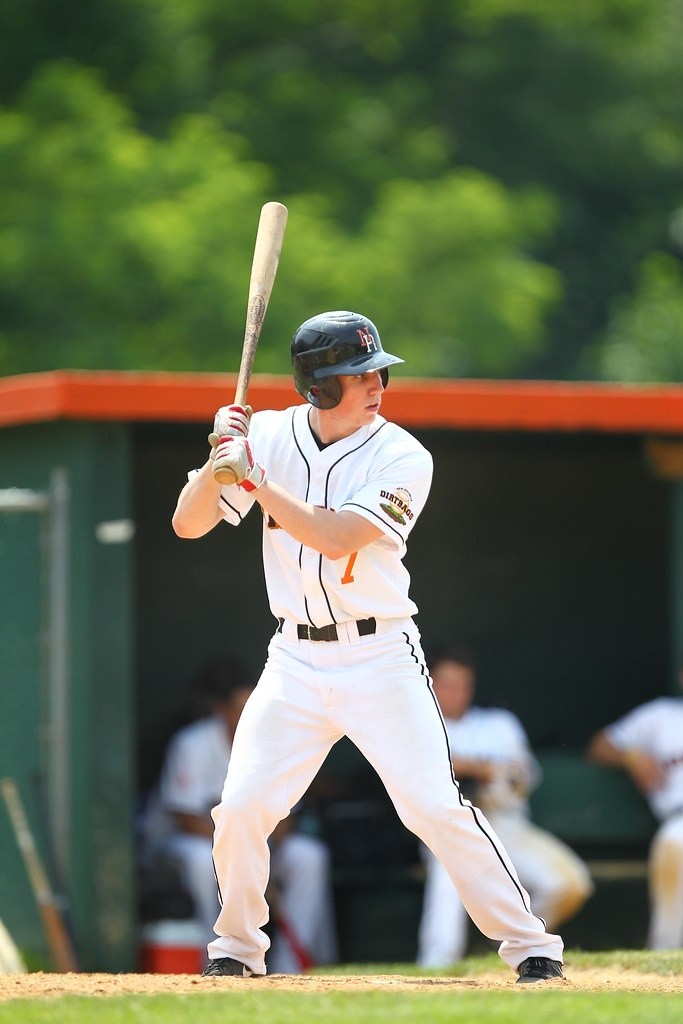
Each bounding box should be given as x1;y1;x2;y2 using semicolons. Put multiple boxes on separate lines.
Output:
214;200;291;487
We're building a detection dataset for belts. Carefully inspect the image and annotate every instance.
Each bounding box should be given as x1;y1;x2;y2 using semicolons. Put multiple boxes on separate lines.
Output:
278;617;376;641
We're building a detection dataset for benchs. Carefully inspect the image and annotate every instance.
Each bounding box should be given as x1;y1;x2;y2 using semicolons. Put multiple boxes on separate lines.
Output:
523;751;658;878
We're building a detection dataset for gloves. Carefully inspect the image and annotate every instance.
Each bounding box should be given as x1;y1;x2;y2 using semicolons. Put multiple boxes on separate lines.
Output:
210;405;253;461
208;433;266;492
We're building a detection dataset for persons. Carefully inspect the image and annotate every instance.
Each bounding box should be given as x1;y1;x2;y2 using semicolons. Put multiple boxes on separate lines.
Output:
410;643;594;977
594;694;683;950
139;655;327;975
168;311;574;987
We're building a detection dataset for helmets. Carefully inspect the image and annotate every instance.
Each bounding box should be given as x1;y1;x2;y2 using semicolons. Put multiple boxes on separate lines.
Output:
291;311;405;409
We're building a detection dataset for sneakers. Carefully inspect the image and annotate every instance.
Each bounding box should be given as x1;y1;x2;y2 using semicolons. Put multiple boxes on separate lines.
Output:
514;957;563;985
202;957;268;978
